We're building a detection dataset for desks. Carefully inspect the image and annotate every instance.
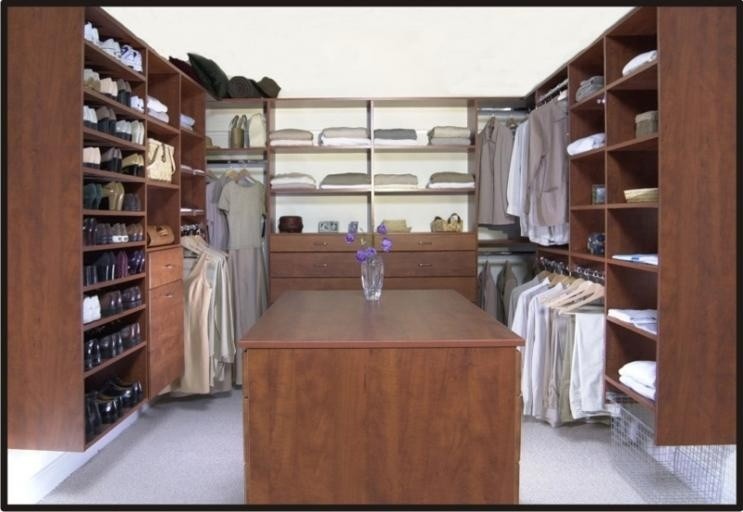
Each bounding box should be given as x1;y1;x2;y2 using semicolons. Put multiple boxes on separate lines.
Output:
238;289;526;505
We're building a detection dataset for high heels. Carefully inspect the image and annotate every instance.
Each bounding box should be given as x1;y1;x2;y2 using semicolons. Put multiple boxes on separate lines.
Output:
84;68;145;286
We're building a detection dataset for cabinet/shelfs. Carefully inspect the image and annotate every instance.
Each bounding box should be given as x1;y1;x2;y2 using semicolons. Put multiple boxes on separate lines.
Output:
148;43;180;254
570;32;605;309
3;4;147;454
605;4;737;445
206;95;536;291
180;70;206;240
146;247;186;400
536;55;569;274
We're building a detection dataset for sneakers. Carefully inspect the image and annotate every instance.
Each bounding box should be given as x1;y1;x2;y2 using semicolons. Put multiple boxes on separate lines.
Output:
85;21;142;72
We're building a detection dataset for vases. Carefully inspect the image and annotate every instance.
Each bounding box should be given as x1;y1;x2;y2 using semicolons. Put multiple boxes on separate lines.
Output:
342;219;393;303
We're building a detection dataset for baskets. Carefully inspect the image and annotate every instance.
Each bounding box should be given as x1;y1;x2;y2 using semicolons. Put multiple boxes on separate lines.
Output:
623;188;658;203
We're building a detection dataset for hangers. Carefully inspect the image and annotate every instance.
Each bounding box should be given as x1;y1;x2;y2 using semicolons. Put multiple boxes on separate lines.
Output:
511;256;605;321
178;222;231;269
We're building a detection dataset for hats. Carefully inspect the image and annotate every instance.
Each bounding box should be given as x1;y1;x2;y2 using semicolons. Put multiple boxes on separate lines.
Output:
251;77;281;98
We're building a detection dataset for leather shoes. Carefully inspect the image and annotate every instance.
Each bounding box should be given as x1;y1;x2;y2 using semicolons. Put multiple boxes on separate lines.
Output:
85;285;143;439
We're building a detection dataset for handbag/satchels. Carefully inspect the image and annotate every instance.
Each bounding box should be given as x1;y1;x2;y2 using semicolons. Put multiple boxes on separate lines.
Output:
147;138;176;182
147;224;175;247
229;113;268;149
431;213;463;232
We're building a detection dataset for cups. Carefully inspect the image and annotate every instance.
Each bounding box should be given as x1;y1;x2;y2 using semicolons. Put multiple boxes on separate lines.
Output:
231;127;244;149
361;260;384;303
